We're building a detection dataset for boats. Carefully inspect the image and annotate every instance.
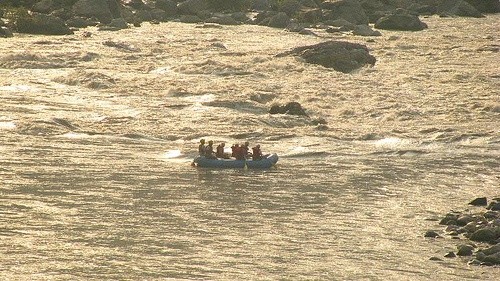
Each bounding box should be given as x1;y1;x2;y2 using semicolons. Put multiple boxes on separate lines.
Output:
194;152;279;169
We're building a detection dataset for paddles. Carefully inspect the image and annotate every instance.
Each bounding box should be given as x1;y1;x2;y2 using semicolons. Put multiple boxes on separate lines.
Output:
242;153;248;172
260;153;280;171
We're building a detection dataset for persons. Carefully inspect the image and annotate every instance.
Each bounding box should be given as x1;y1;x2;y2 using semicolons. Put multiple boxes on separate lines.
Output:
236;147;247;160
232;145;239;156
243;142;253;158
253;145;263;160
199;139;205;156
205;140;215;158
217;143;225;156
